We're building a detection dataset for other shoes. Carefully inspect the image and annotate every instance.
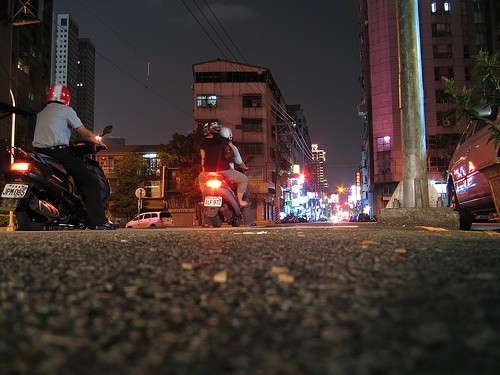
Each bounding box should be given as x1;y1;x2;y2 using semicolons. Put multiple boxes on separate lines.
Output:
240;201;252;208
250;223;257;226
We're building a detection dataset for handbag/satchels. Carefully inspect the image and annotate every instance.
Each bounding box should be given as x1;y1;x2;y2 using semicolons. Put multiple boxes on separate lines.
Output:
223;145;234;163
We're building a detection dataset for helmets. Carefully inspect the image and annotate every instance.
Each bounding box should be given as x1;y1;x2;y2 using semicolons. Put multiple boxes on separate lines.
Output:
47;84;71;106
203;122;219;135
220;127;232;140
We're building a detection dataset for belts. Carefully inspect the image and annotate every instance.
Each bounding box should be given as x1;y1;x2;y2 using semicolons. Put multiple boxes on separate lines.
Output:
50;145;66;150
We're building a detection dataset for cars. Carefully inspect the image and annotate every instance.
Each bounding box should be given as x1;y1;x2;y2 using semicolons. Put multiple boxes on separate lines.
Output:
125;210;175;229
279;209;374;223
445;98;500;231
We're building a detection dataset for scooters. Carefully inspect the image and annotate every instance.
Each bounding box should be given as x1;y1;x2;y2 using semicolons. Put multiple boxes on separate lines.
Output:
201;168;252;228
1;124;113;231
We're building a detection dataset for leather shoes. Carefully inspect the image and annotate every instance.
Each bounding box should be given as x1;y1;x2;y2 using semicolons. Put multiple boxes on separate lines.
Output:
91;223;117;230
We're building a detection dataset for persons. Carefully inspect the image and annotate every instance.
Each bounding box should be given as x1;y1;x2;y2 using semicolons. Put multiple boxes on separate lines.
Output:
202;127;257;228
32;84;118;230
199;122;248;206
